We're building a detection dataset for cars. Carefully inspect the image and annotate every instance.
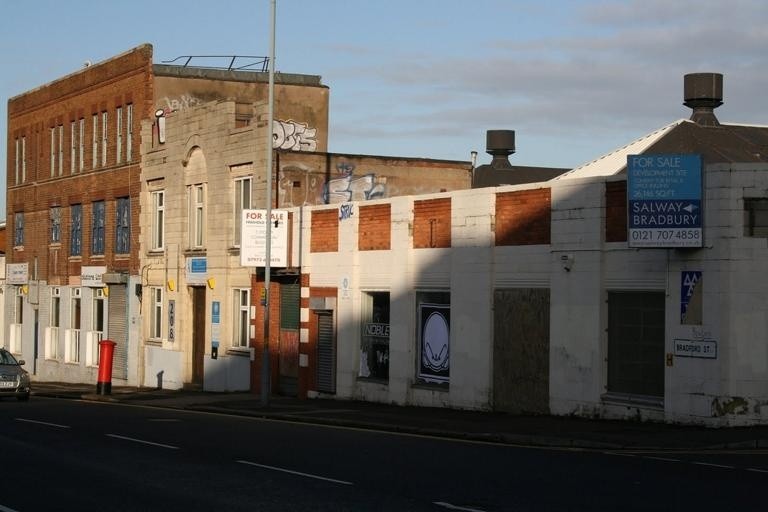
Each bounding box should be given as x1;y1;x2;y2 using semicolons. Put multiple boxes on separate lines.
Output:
0;348;31;400
